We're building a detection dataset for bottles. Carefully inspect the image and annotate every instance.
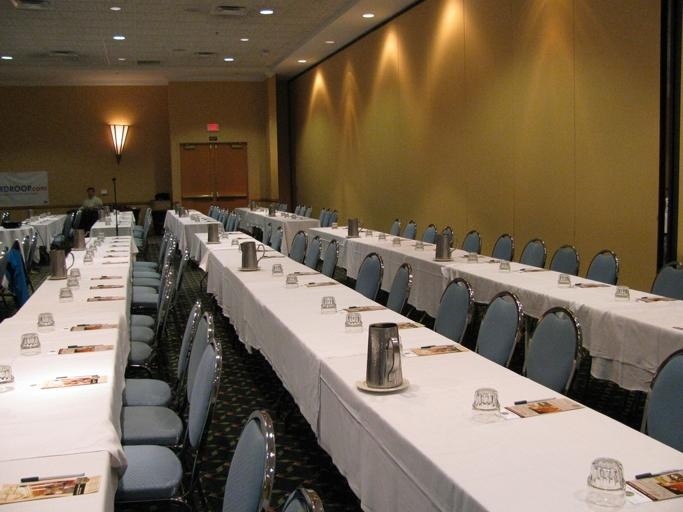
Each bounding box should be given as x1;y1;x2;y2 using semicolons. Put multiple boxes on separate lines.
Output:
347;219;457;260
205;223;403;388
50;229;90;278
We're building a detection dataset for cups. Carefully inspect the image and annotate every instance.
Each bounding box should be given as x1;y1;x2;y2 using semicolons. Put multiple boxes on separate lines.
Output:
281;213;296;220
0;267;81;394
104;222;111;226
366;230;424;251
191;213;200;222
468;252;630;303
472;388;501;423
30;212;51;224
222;233;239;248
272;264;363;334
332;222;338;229
588;456;625;508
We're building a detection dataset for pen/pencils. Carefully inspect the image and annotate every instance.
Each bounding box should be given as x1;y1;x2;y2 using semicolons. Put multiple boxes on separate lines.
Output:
514;398;555;405
21;472;85;482
68;345;91;348
636;469;680;479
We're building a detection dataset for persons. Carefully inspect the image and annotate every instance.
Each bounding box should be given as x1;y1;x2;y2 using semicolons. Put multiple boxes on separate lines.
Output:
82;187;103;237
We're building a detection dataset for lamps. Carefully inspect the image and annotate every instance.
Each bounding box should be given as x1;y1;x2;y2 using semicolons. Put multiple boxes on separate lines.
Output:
107;123;128;164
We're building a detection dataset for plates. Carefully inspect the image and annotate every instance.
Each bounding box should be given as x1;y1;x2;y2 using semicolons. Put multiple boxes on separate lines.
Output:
357;377;409;393
